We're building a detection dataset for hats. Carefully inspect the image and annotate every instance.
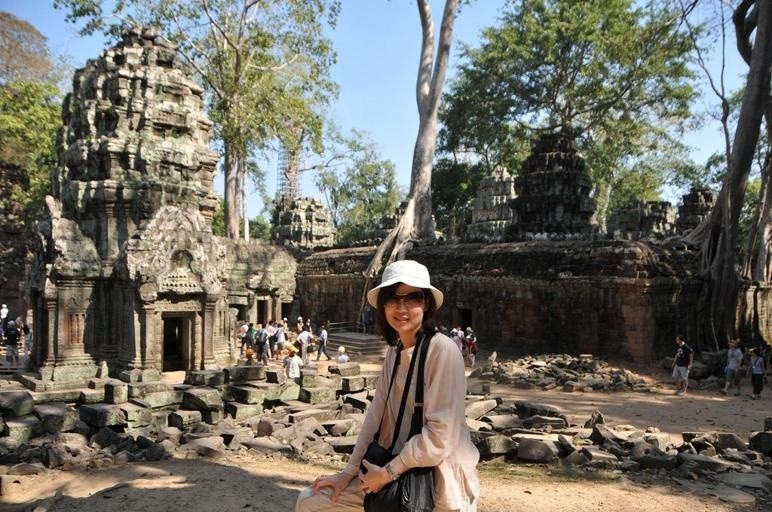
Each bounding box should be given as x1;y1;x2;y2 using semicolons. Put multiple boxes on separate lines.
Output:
246;349;255;354
287;346;300;354
366;259;445;311
297;316;303;321
449;328;459;334
466;327;473;332
337;345;346;355
728;339;737;346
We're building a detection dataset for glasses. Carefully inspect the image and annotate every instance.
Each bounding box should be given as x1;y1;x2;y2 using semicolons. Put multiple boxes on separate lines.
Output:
381;291;427;310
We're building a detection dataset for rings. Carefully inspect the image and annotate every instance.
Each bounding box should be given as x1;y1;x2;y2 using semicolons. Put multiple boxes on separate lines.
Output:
366;489;371;494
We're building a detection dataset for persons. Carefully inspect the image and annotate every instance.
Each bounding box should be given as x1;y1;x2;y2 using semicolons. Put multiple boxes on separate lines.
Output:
426;317;478;368
735;337;772;383
0;304;33;365
336;347;350;363
671;335;694;396
239;316;312;365
281;345;304;385
362;305;380;335
314;325;332;361
720;339;744;396
295;258;482;512
746;348;766;399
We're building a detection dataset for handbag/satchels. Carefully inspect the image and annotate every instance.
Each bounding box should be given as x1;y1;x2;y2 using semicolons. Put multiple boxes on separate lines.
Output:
469;342;479;354
358;439;439;512
307;343;313;353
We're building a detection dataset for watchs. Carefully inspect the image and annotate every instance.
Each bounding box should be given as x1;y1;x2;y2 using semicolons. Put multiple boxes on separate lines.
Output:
386;463;402;482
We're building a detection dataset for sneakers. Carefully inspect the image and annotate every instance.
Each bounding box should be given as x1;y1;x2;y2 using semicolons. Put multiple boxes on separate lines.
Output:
719;389;742;396
675;389;688;396
750;394;762;400
